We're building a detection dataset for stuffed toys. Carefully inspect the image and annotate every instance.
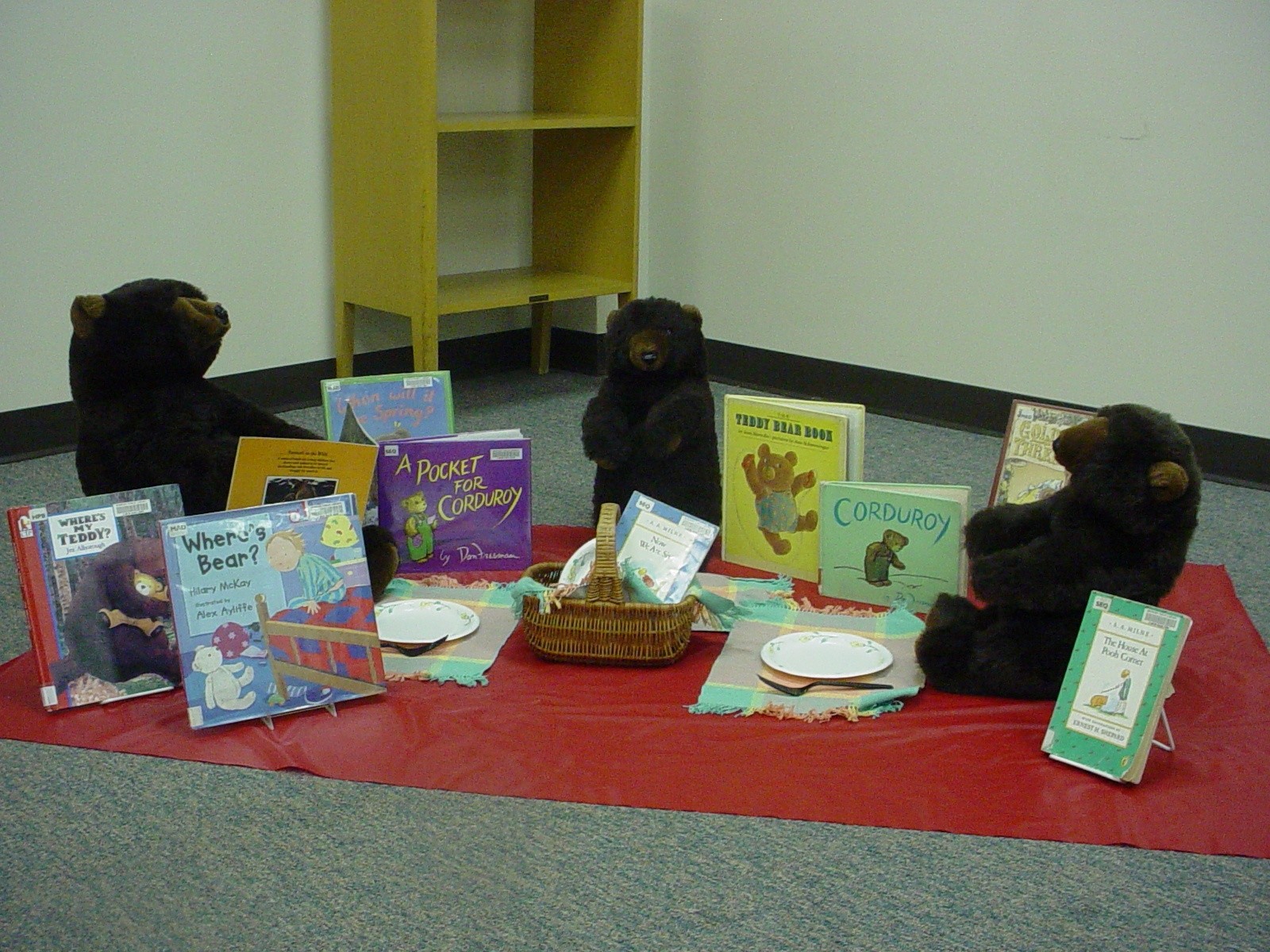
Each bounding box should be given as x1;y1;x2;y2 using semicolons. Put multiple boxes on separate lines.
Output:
583;298;723;530
911;403;1201;701
68;277;401;597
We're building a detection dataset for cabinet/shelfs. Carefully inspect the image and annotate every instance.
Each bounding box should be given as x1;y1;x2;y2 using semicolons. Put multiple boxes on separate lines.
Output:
322;0;644;378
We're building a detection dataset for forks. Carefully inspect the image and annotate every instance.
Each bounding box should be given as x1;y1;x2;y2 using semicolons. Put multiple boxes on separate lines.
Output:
380;635;448;657
756;673;894;697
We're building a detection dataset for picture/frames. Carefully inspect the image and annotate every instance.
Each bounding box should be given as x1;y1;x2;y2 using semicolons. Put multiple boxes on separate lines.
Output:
988;399;1096;506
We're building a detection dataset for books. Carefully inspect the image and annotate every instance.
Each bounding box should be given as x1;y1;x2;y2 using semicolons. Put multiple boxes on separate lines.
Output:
612;490;720;606
321;369;455;530
379;426;532;572
720;392;972;616
1039;588;1194;785
985;399;1100;509
224;402;381;528
7;481;384;732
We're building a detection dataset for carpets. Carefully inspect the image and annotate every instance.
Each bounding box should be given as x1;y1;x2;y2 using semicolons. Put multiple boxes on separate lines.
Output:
0;524;1270;864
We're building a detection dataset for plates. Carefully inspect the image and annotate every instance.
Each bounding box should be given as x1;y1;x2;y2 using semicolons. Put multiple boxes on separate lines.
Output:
557;538;596;590
373;598;480;643
761;631;893;679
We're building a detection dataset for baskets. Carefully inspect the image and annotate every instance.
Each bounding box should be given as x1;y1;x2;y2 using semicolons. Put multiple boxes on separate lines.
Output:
519;503;700;667
